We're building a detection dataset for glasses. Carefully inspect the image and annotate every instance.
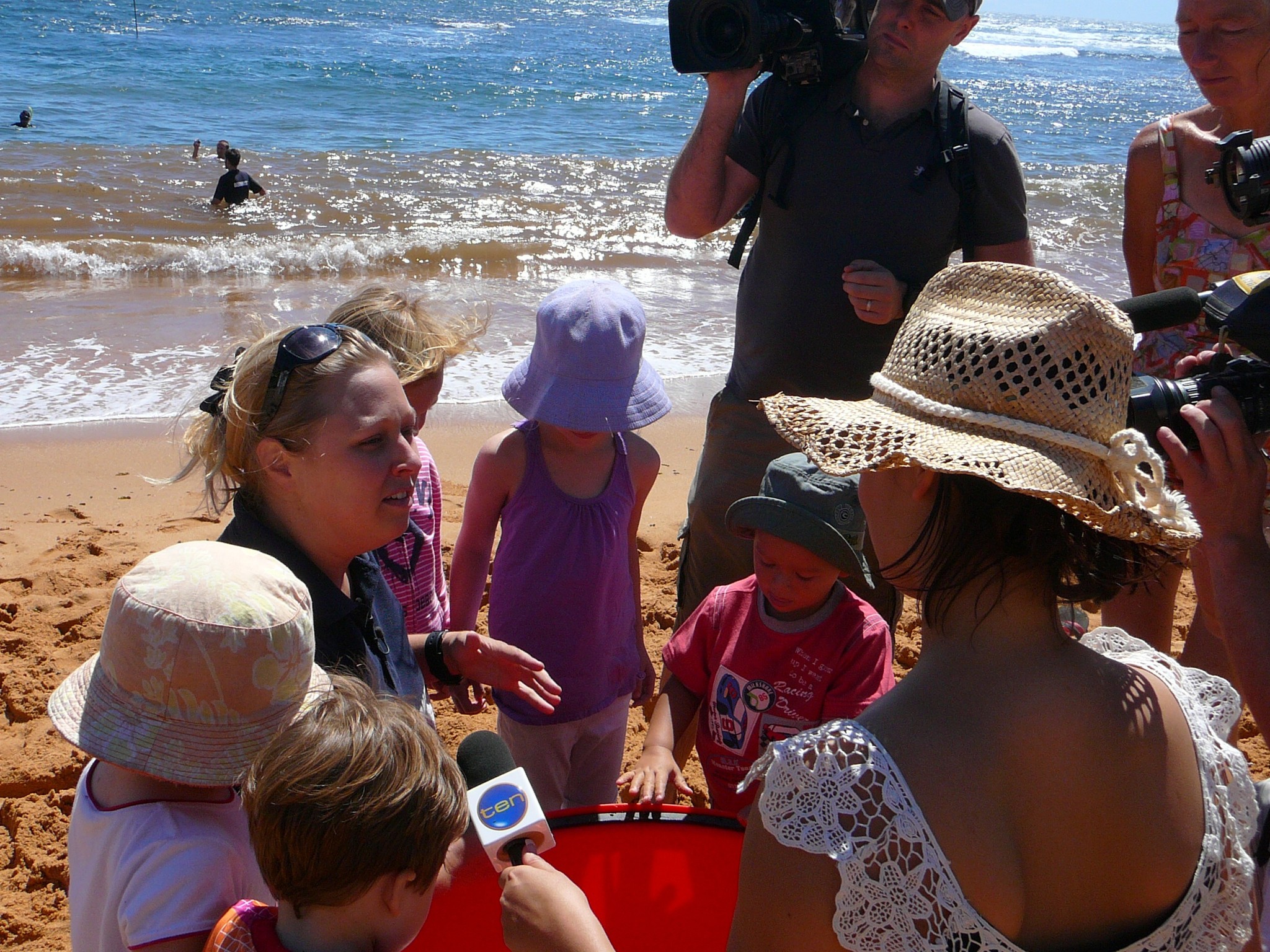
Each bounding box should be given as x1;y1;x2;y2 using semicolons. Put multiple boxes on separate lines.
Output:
22;116;31;121
261;320;352;437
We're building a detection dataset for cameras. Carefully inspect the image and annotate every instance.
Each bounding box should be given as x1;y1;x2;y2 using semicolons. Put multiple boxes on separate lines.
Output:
1203;130;1270;226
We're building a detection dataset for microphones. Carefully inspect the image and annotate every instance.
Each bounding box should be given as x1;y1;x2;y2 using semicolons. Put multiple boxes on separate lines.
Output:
1112;280;1225;336
455;731;557;874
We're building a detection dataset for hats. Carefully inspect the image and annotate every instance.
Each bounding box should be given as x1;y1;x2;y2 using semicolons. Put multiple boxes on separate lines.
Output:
501;282;674;433
751;259;1204;562
725;450;873;590
927;0;983;19
45;540;336;788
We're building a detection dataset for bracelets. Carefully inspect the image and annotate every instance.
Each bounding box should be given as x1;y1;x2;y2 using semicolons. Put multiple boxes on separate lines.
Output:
423;629;464;687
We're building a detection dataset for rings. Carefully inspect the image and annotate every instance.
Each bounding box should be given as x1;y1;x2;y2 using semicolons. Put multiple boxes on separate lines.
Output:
866;299;872;312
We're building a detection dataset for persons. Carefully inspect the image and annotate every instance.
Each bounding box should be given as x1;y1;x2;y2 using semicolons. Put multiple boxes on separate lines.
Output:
614;451;899;819
447;268;657;817
497;853;620;951
660;0;1036;805
191;138;230;159
1094;335;1270;763
10;110;36;129
317;283;455;701
134;319;567;736
198;671;475;952
210;148;266;208
720;256;1263;952
45;538;337;952
1094;0;1270;662
